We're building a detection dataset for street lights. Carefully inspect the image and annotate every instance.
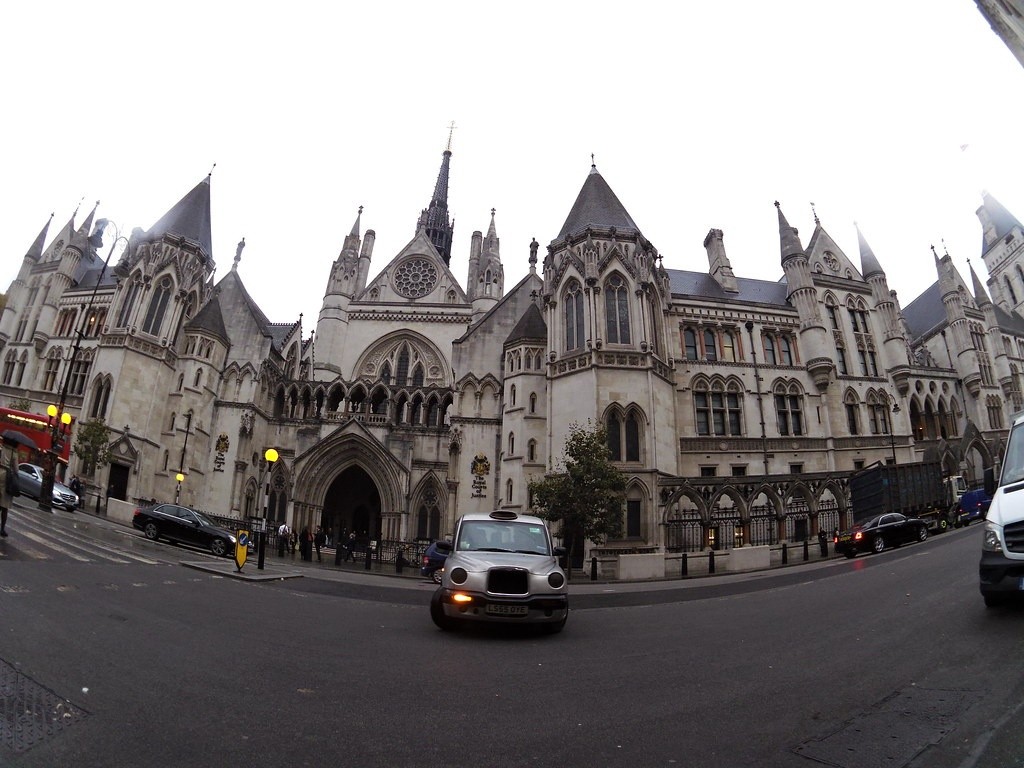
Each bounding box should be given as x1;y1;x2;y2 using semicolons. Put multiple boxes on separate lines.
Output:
174;473;184;505
886;393;902;463
37;405;72;513
256;449;278;569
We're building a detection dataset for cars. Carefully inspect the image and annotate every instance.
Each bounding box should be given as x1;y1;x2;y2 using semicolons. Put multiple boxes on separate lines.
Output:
422;540;453;583
956;488;992;527
834;512;928;558
131;504;253;557
17;463;79;512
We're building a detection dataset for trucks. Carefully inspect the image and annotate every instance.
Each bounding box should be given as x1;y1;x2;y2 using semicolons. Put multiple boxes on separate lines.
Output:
849;460;970;536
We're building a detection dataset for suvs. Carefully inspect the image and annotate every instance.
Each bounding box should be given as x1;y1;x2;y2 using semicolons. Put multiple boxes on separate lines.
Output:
431;511;568;633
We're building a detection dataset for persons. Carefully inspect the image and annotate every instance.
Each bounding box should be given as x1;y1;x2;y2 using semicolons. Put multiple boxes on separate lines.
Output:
344;533;357;563
831;527;840;554
0;437;19;537
106;485;114;510
312;526;323;562
68;475;86;510
278;521;298;554
817;527;828;558
299;527;313;562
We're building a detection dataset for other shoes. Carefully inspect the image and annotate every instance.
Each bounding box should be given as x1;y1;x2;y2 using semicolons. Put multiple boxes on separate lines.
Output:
315;559;321;562
0;531;8;536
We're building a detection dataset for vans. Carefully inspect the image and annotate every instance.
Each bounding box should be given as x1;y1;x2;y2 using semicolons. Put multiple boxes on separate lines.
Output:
979;410;1024;607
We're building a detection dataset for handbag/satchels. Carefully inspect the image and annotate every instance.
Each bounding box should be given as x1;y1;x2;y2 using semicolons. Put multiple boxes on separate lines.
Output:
81;486;86;496
6;470;21;497
282;531;288;538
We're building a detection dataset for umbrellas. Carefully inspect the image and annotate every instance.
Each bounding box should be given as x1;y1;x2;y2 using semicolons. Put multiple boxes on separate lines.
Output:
0;429;37;450
